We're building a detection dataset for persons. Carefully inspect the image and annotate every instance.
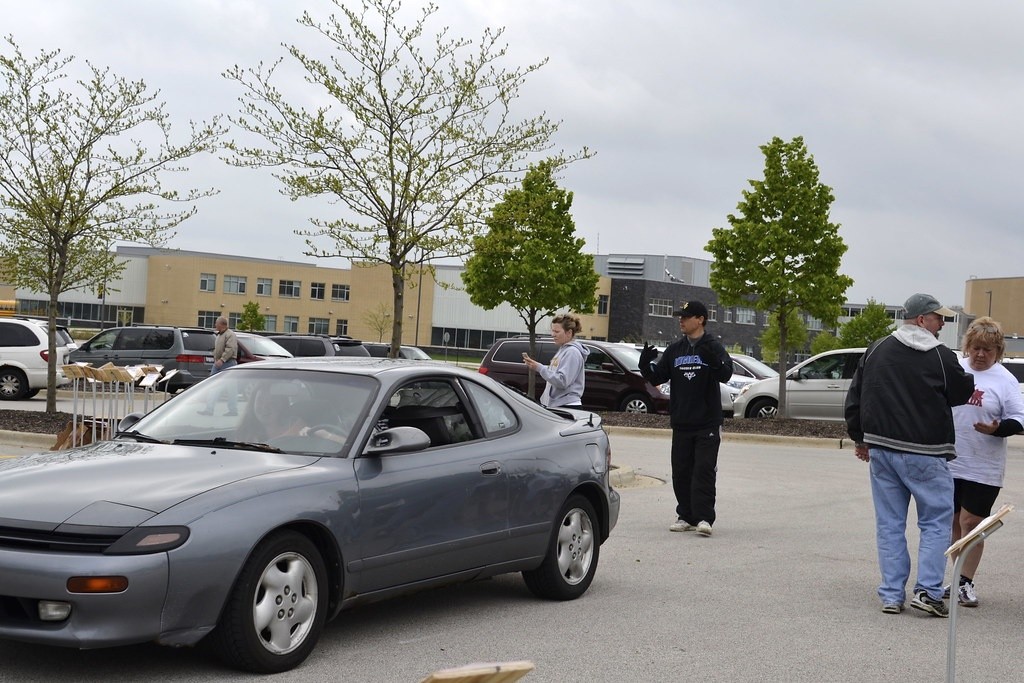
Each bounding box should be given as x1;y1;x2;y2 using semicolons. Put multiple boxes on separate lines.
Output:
210;316;238;377
637;301;734;537
522;314;591;410
843;293;977;618
942;315;1024;607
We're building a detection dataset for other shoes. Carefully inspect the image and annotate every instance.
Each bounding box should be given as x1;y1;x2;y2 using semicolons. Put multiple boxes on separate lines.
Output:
223;411;238;416
197;411;214;415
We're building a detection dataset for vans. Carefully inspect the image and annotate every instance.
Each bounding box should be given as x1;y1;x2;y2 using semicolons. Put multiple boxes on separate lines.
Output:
612;341;867;420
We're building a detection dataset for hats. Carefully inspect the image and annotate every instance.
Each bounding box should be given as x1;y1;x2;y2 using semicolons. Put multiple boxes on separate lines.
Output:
900;292;957;321
672;300;708;320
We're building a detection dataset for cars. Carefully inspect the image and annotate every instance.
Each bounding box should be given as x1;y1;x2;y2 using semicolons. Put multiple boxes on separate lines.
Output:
231;330;433;402
2;356;622;674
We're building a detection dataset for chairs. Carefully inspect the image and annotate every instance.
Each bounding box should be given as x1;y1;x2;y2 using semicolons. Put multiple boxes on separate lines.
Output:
289;400;340;435
125;340;137;349
388;405;453;446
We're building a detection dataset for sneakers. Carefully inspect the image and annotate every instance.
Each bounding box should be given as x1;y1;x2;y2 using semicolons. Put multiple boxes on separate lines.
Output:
669;520;696;531
882;604;906;614
695;520;712;536
957;585;979;607
941;585;950;599
910;589;950;617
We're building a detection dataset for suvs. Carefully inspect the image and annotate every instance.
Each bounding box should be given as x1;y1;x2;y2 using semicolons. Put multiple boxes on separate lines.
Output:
478;334;673;414
70;323;220;393
0;315;79;401
